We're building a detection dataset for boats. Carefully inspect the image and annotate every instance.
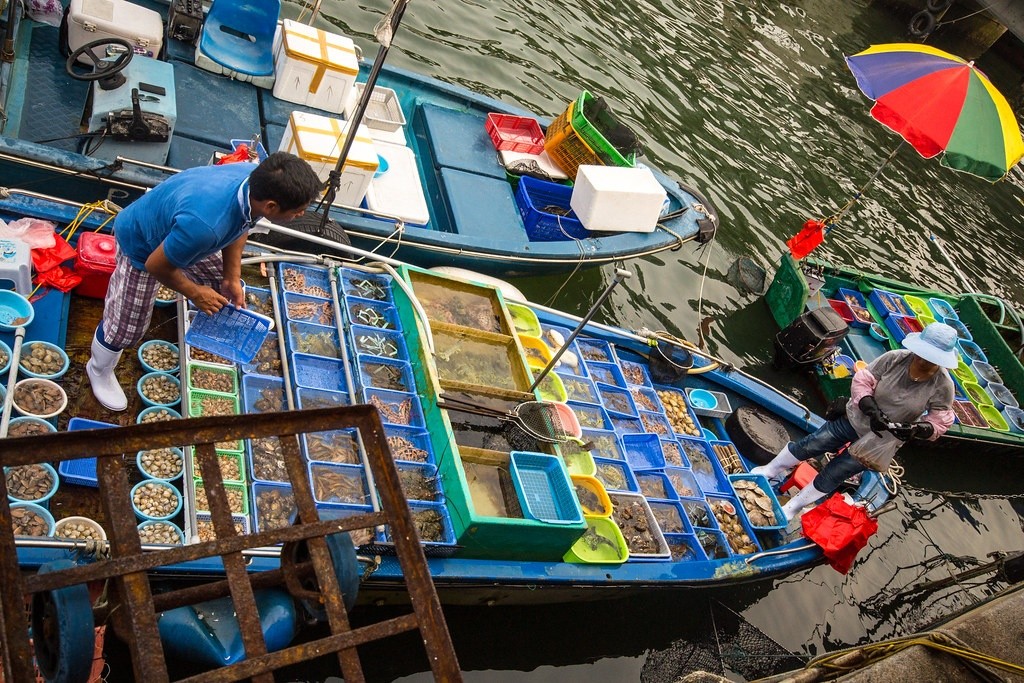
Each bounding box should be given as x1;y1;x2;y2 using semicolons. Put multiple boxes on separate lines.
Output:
0;185;907;633
765;252;1024;473
1;1;720;277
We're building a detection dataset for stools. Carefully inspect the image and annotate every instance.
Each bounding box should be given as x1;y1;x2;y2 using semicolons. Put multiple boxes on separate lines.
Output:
0;237;35;298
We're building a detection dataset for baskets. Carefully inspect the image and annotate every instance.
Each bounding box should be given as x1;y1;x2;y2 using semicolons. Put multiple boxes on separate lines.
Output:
0;263;456;546
829;288;1024;435
506;302;789;563
484;111;545;154
232;139;268;164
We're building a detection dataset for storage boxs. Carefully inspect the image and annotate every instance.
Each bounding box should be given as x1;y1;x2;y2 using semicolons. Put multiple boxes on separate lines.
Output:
515;174;591;241
542;89;637;182
277;110;379;208
66;0;164;67
569;163;666;233
272;17;359;114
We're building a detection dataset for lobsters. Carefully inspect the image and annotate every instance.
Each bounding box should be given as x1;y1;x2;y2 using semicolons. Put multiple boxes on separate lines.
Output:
369;394;446;542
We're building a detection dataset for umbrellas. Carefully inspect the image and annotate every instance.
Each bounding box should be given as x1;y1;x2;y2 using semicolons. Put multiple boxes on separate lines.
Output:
825;43;1024;237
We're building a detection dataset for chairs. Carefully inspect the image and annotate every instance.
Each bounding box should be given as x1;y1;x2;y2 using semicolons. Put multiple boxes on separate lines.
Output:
194;0;282;89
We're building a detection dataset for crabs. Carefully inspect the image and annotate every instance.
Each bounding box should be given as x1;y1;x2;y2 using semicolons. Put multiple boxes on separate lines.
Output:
284;268;335;326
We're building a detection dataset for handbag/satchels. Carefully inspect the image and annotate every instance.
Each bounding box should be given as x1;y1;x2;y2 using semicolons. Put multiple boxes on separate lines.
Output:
849;428;901;472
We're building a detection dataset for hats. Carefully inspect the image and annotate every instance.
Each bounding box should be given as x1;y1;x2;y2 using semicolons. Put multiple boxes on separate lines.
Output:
901;323;958;369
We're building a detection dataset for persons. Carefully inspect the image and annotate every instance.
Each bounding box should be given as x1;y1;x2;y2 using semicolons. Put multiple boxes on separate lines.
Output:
751;321;958;523
86;150;321;411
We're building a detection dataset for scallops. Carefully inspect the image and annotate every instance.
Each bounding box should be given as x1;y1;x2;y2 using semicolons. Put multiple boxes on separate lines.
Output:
733;478;778;528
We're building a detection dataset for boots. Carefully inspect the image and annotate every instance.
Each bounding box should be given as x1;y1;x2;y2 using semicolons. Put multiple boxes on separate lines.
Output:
85;326;128;411
751;441;802;480
782;477;828;522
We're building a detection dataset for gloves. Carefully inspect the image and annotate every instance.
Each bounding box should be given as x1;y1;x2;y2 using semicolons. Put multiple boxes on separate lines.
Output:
888;421;934;442
858;396;892;438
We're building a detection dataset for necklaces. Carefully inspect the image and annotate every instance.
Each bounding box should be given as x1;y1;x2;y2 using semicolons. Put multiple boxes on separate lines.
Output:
910;374;921;383
